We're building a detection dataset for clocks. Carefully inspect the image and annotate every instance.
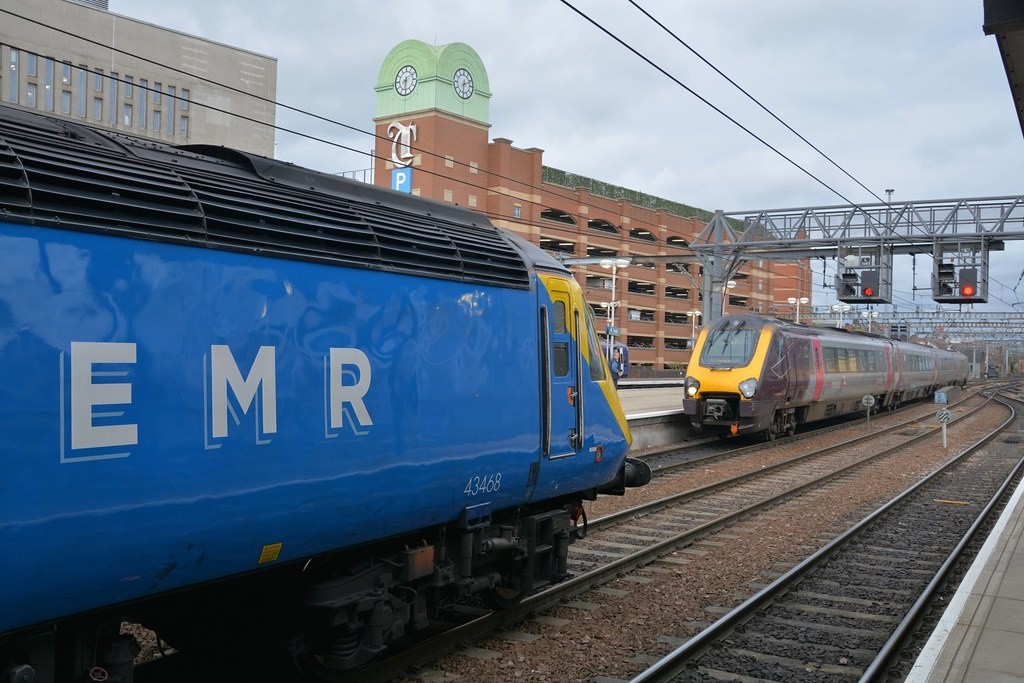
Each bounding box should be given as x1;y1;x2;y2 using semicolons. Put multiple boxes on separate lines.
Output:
395;65;417;96
454;68;474;99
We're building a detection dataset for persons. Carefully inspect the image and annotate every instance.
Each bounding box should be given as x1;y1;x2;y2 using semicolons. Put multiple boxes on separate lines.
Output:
608;352;624;391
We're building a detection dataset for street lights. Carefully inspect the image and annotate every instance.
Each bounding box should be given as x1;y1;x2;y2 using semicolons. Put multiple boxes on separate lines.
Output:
686;311;702;350
599;259;630;357
788;298;810;324
601;302;618;359
833;305;850;328
861;312;879;333
722;281;737;313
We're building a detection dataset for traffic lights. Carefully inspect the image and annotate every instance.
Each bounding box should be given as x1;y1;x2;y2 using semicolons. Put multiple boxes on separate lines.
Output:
860;270;879;298
958;269;977;297
842;284;855;296
941;283;952;295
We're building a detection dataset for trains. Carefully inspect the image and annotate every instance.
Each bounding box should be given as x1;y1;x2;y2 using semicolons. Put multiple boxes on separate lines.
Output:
682;310;973;443
1;106;655;683
